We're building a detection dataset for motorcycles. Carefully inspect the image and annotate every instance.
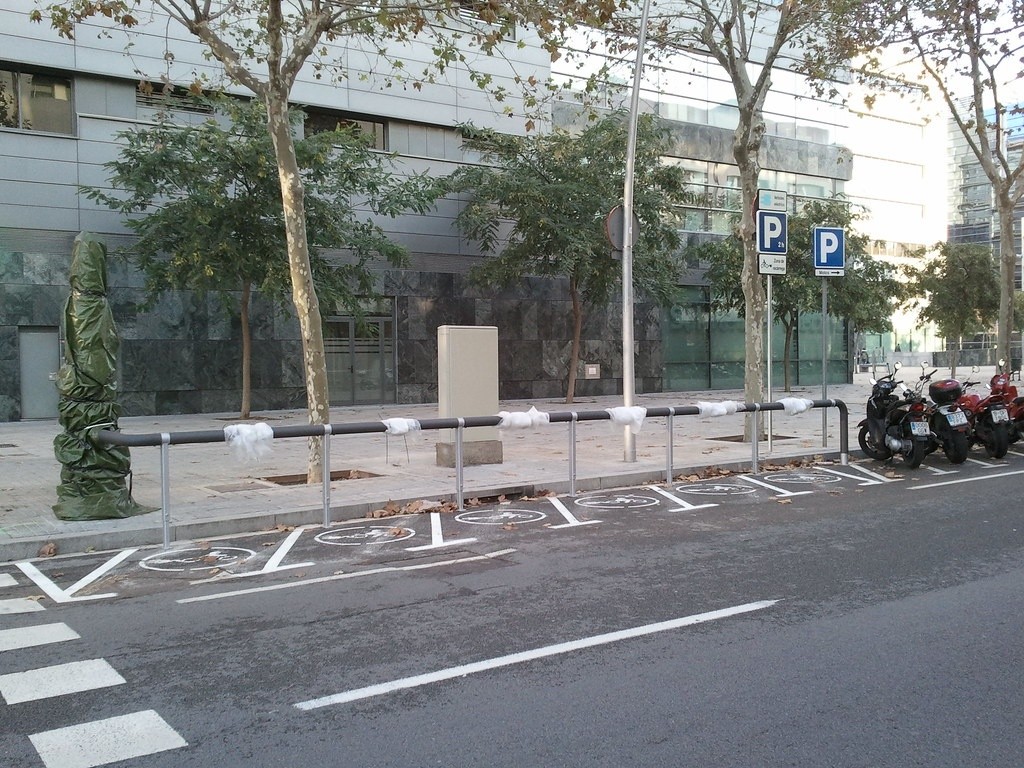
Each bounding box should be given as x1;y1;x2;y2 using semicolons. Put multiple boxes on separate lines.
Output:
856;360;1023;467
